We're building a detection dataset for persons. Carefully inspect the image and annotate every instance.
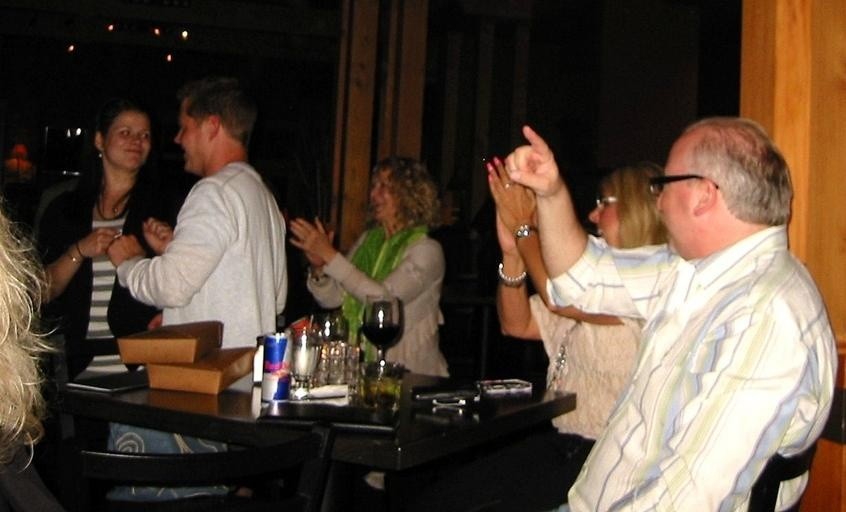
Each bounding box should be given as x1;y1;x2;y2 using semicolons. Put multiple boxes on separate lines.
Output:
505;115;838;512
0;202;80;512
103;72;287;395
31;102;191;512
290;156;450;381
464;156;678;492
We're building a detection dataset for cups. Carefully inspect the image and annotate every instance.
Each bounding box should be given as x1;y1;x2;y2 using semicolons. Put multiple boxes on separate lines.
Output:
363;296;404;365
292;330;320;388
319;342;361;384
360;360;401;413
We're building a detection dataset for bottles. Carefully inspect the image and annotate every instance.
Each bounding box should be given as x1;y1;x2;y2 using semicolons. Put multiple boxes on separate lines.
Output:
262;335;289;400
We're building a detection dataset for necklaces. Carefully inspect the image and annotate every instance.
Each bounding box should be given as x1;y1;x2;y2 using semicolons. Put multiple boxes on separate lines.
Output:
103;172;136;218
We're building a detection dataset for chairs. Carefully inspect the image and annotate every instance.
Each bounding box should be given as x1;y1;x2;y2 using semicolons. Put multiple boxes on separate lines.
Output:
747;439;819;512
54;417;338;512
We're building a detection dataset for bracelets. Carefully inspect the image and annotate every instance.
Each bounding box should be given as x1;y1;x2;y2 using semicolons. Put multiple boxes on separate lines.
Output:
499;262;529;288
305;268;332;283
65;243;85;264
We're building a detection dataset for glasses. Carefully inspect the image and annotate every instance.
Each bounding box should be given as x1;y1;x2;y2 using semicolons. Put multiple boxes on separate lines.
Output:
595;195;618;206
647;173;719;192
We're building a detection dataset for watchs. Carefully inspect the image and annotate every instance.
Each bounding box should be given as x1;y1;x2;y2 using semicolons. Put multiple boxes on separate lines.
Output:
512;224;536;241
